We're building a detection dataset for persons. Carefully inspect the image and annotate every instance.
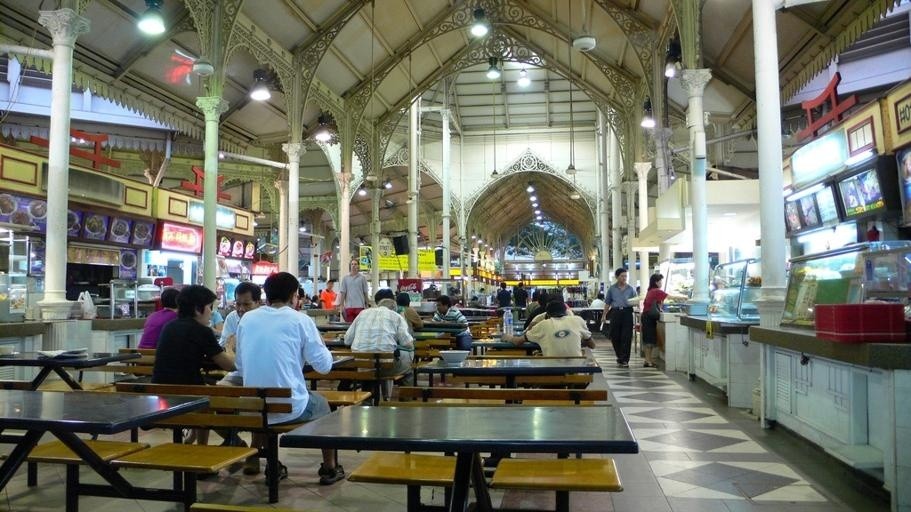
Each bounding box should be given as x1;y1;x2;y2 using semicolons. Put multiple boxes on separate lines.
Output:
151;283;238;480
296;280;337;321
210;280;267;476
210;306;225;336
602;267;638;369
333;258;373;325
503;292;597;389
344;290;423;400
139;289;179;348
640;272;690;369
590;294;605;310
433;294;471;351
470;288;485;309
232;272;346;487
495;283;529;320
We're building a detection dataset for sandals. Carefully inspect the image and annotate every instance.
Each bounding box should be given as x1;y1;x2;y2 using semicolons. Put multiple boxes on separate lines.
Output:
643;362;658;368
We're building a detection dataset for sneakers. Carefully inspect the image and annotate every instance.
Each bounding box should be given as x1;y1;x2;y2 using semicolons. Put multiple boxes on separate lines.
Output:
619;361;629;368
219;439;249;448
242;456;260;475
318;462;345;485
264;461;288;486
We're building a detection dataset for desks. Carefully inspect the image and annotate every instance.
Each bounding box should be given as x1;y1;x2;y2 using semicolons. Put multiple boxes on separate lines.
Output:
280;404;639;511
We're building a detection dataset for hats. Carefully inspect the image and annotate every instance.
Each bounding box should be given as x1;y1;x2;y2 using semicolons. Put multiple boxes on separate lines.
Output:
545;300;566;317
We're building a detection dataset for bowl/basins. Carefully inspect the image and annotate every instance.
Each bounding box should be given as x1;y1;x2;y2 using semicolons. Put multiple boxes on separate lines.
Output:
441;351;469;367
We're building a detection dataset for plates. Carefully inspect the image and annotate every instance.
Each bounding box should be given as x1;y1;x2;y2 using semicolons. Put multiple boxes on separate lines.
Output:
0;193;153;270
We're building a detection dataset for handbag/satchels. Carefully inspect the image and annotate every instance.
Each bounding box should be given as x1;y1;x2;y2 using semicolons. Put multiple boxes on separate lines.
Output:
648;298;660;320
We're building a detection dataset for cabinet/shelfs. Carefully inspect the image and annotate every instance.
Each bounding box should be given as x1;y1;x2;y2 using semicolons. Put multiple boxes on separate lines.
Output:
654;240;911;329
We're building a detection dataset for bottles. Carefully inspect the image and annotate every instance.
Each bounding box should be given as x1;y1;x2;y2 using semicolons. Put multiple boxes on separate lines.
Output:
502;307;514;335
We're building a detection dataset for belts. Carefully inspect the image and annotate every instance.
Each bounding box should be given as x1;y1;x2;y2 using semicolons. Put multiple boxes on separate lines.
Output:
614;307;632;310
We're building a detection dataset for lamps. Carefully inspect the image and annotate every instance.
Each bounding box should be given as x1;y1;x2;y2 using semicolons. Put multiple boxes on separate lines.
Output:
566;0;579;177
486;57;503;179
249;69;271;105
469;9;490;39
132;1;171;37
